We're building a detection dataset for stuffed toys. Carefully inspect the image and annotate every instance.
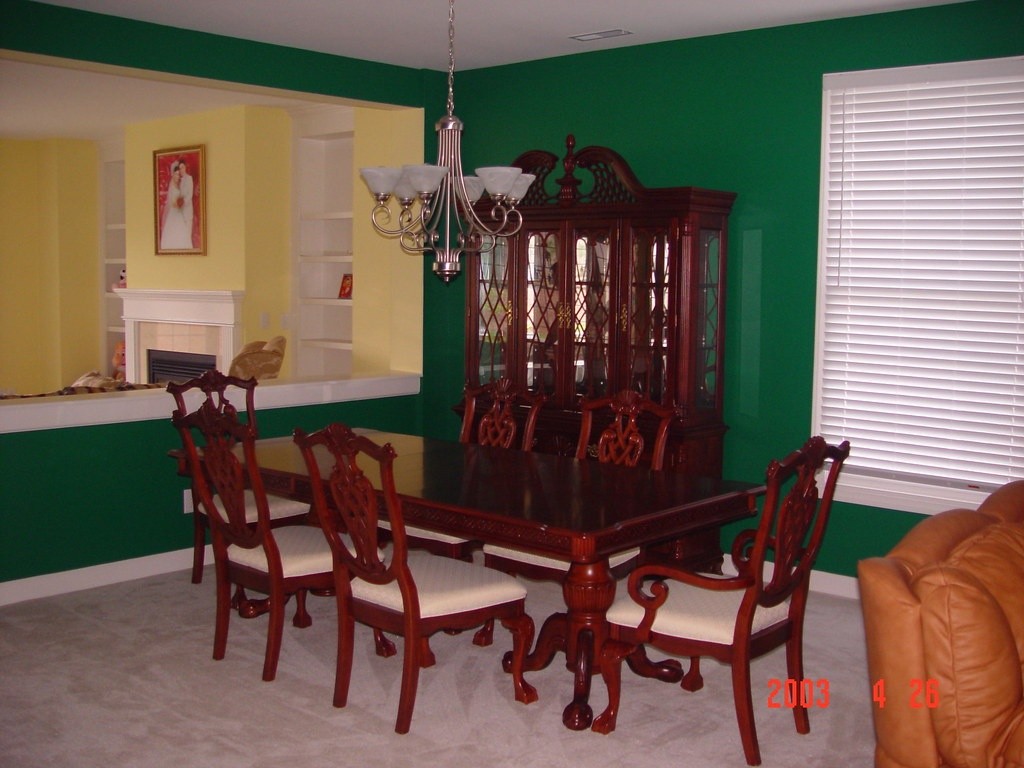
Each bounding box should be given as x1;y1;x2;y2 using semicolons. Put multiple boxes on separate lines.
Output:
119;269;126;288
110;339;126;381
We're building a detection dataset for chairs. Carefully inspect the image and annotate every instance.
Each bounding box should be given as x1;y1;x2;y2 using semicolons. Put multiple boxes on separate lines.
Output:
226;336;286;380
166;369;310;585
293;424;540;734
594;435;853;766
471;387;684;647
172;398;396;685
377;373;550;646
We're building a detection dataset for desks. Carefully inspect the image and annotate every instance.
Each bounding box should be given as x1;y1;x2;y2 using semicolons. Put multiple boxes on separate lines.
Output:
175;428;768;730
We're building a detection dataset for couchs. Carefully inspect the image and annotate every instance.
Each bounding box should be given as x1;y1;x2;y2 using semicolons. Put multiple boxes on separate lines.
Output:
857;480;1024;768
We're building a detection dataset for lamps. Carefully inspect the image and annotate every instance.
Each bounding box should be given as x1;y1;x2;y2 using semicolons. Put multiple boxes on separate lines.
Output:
358;0;538;283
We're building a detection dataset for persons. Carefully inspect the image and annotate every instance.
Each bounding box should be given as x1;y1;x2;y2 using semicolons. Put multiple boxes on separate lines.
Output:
161;160;194;249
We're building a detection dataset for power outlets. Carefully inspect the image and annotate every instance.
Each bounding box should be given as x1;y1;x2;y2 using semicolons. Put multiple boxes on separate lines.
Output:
184;489;194;514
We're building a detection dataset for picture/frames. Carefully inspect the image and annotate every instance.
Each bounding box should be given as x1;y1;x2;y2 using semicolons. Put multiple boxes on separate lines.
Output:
151;143;206;256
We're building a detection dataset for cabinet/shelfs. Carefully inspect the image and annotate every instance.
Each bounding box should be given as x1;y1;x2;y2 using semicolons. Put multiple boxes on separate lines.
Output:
289;129;355;376
444;133;737;572
100;159;126;379
